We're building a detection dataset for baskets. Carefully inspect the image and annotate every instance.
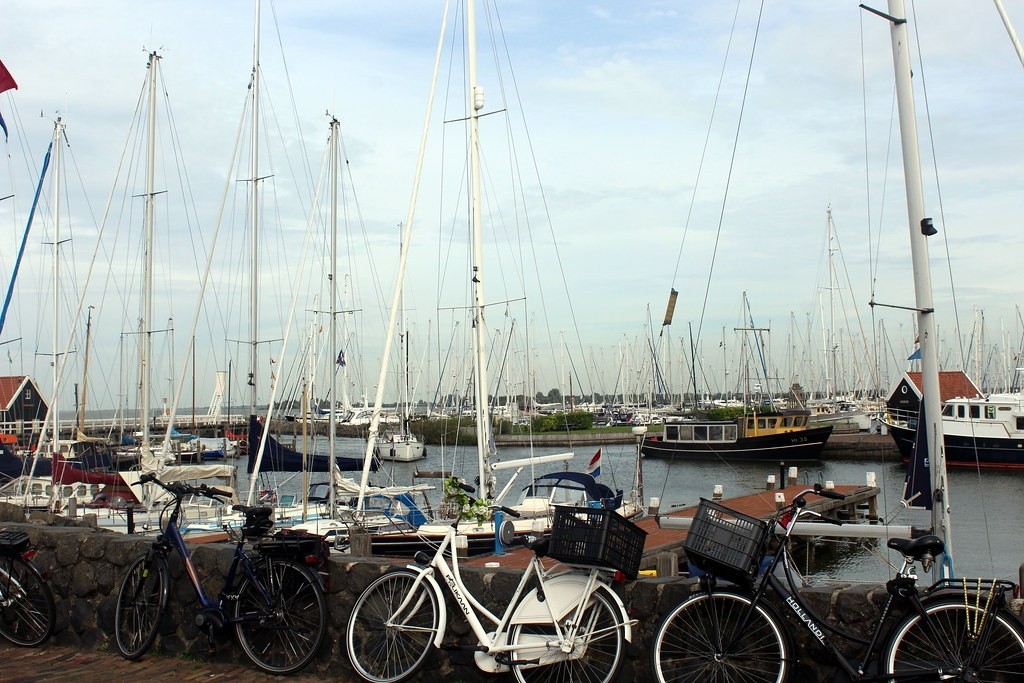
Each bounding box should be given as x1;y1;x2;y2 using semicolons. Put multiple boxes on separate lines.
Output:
549;503;650;582
682;496;767;587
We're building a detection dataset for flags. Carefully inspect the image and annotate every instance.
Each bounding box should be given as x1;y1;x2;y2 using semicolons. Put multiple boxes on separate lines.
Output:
588;450;600;480
337;351;347;366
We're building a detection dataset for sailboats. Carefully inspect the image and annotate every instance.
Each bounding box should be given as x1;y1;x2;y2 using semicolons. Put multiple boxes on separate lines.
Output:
1;3;1024;559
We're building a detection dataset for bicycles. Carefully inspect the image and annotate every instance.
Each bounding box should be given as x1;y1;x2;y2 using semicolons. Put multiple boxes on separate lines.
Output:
647;482;1024;682
0;531;58;648
343;493;649;682
114;473;331;675
